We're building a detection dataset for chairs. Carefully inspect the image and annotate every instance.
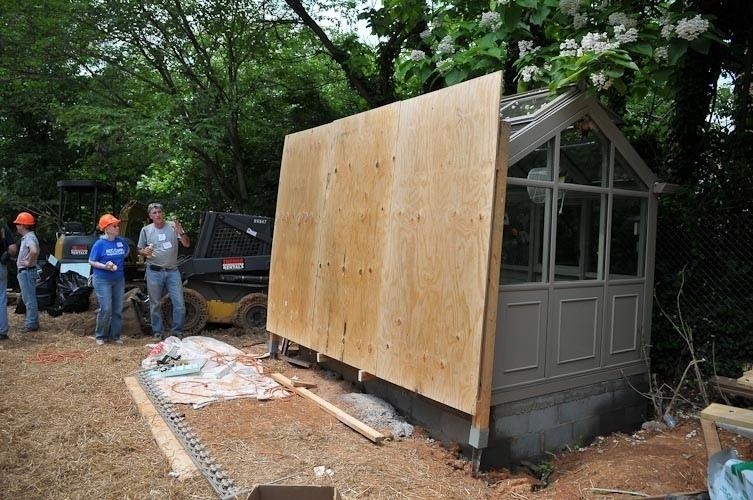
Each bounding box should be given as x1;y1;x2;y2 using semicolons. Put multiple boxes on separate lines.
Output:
62;221;86;237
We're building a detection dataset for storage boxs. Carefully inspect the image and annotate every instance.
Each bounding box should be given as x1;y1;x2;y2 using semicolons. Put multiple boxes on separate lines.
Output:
245;483;342;500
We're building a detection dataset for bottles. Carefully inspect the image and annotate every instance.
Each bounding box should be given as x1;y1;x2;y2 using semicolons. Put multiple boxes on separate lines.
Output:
145;244;153;259
663;413;676;428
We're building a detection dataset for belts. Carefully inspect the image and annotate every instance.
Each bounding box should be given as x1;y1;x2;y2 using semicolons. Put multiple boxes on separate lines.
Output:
160;267;173;272
17;265;37;271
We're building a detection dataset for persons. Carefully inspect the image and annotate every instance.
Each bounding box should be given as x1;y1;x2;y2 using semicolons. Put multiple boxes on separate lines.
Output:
7;240;19;290
136;202;192;343
88;212;130;346
0;219;18;341
12;212;42;334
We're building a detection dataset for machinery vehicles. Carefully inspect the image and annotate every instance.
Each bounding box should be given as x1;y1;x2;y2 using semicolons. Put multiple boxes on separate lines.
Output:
71;210;274;341
36;178;152;313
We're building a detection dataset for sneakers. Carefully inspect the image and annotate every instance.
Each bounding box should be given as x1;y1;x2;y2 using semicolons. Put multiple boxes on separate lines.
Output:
0;334;9;341
111;339;124;345
95;338;105;347
174;332;186;341
20;324;40;333
151;332;166;342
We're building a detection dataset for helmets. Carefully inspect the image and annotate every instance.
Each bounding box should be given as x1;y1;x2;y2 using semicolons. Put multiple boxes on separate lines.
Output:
98;213;122;232
12;211;36;226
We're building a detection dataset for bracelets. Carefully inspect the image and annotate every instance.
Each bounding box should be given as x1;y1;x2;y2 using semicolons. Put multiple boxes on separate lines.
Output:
179;229;186;236
